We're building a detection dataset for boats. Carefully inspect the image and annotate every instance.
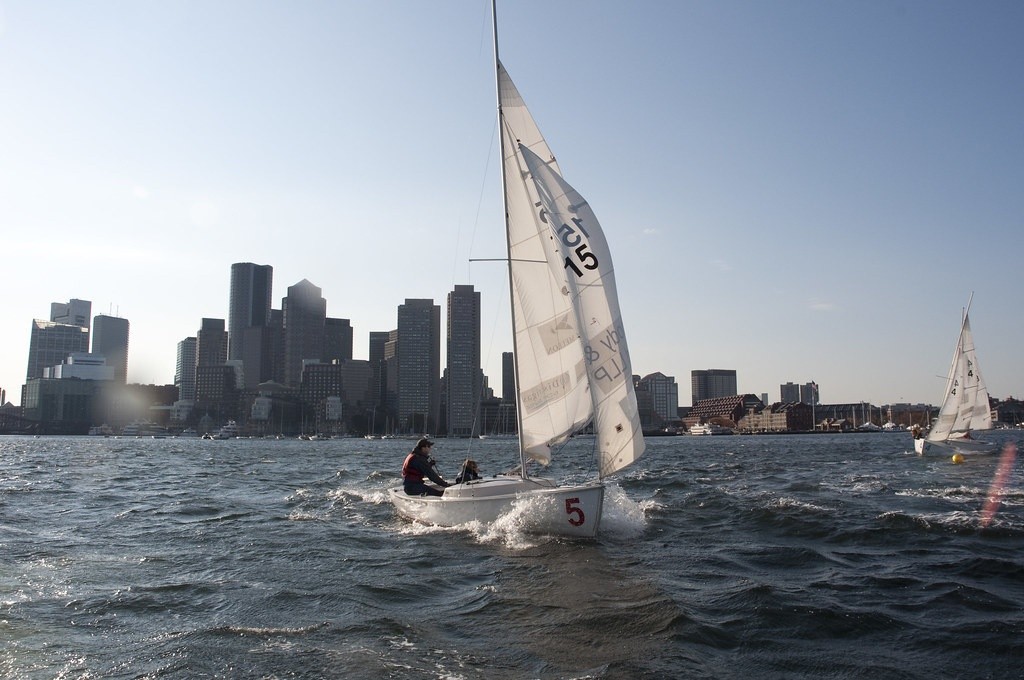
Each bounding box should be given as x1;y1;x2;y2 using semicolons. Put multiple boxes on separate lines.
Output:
690;422;723;436
87;419;520;441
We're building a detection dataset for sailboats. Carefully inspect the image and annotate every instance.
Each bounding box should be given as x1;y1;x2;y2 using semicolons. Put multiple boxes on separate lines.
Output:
912;291;1000;459
386;1;649;545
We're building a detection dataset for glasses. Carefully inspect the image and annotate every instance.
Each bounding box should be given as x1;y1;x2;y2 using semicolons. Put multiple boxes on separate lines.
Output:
472;466;477;468
425;445;430;448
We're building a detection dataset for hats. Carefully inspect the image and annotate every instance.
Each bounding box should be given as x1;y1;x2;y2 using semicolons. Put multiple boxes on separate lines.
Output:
416;438;434;449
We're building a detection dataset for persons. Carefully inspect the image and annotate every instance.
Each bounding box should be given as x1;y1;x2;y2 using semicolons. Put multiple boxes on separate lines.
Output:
457;459;482;484
912;429;924;439
401;439;452;497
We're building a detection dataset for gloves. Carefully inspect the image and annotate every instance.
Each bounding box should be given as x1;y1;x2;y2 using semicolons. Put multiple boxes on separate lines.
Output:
430;459;435;465
